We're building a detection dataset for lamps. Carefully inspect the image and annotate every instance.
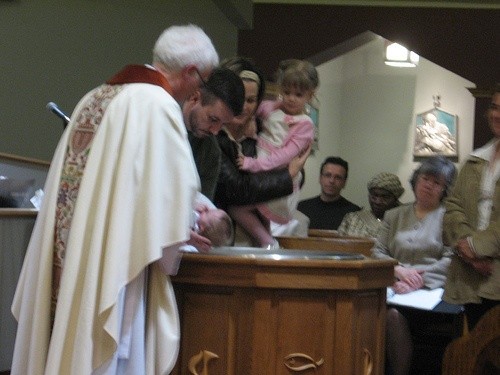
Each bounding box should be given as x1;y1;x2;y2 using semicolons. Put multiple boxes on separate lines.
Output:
385;42;420;68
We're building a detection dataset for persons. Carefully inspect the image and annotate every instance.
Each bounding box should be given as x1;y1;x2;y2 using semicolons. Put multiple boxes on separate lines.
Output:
295;155;360;231
186;62;245;255
440;85;499;333
186;184;235;249
337;170;408;238
228;57;322;250
215;65;313;212
372;154;465;372
8;21;220;374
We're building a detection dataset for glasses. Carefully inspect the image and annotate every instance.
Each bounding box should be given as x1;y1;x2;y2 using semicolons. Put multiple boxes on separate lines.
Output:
193;65;210;90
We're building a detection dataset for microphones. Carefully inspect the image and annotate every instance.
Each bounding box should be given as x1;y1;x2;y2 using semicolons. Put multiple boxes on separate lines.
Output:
46;102;71;123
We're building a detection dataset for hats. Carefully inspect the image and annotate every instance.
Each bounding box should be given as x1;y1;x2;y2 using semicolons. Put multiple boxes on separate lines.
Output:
368;170;404;200
